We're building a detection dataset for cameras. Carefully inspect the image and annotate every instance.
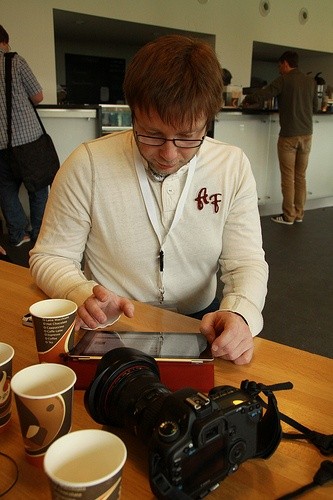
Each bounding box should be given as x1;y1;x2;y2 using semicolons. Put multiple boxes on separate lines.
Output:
83;347;261;500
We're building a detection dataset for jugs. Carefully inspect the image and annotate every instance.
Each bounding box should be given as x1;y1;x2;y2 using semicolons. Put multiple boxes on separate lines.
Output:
314;71;325;111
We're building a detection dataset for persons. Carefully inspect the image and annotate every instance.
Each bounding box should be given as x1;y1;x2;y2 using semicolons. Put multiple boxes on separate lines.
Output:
242;49;314;226
27;34;269;366
0;25;61;248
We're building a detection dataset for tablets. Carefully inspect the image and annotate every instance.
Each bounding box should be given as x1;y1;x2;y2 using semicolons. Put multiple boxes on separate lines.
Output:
67;325;214;362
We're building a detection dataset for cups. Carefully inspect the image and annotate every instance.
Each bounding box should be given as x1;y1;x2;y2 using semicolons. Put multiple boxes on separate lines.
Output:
29;299;78;369
0;342;15;428
10;363;78;467
42;429;127;500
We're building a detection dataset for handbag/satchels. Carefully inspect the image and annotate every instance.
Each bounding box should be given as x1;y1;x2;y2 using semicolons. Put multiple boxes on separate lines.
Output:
9;133;60;195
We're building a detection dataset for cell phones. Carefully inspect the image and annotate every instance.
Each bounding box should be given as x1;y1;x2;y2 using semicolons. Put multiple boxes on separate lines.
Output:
21;313;35;329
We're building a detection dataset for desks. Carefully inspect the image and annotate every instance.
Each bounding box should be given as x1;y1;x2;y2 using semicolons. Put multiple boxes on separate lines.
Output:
0;259;333;500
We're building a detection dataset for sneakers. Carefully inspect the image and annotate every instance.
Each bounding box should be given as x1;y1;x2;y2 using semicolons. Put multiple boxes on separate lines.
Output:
270;213;302;225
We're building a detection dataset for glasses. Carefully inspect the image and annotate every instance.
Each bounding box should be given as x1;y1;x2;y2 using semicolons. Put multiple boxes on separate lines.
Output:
7;43;11;50
133;118;211;149
